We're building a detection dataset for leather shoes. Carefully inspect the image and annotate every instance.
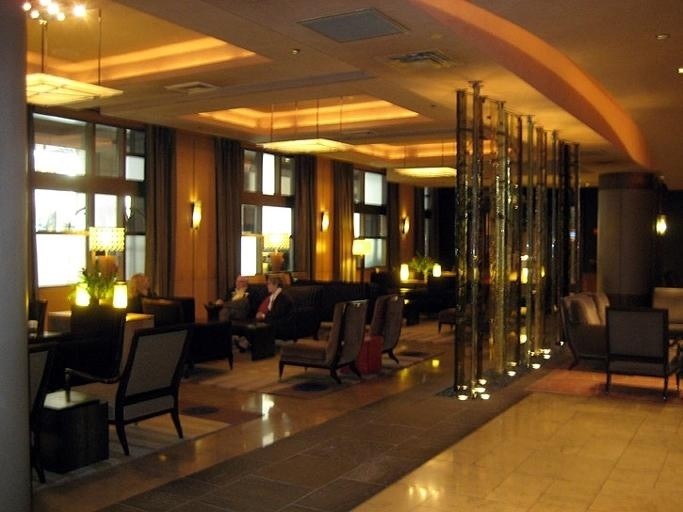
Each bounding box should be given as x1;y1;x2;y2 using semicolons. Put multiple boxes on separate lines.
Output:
234;338;247;354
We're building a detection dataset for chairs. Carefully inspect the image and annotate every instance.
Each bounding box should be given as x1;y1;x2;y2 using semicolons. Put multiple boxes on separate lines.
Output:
557;284;682;401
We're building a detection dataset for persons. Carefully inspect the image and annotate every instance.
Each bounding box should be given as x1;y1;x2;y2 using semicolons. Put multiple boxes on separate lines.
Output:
130;273;152;311
232;276;295;351
213;275;246;324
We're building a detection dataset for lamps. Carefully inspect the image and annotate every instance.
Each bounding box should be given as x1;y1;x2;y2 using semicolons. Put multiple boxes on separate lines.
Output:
255;94;355;157
87;225;126;257
393;134;458;179
21;0;125;113
